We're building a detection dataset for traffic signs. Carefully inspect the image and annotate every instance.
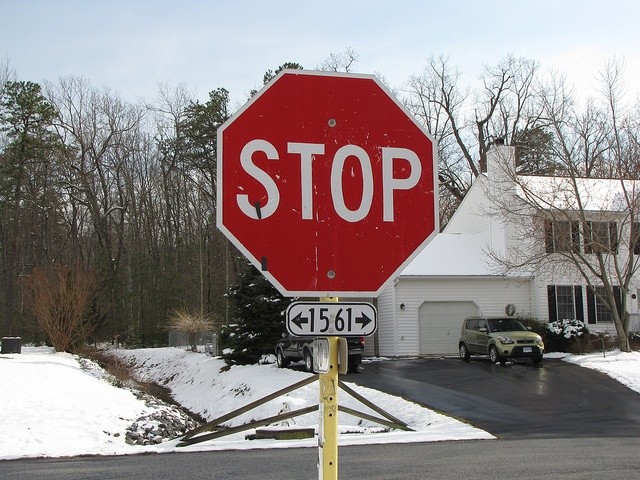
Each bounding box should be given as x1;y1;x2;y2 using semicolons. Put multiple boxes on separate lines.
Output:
284;303;377;337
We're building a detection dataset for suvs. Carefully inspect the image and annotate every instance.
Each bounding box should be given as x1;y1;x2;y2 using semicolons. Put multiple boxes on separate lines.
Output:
275;330;364;371
459;317;545;362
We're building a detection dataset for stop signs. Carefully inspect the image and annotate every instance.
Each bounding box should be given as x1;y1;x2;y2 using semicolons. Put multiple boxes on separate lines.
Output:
217;69;439;297
